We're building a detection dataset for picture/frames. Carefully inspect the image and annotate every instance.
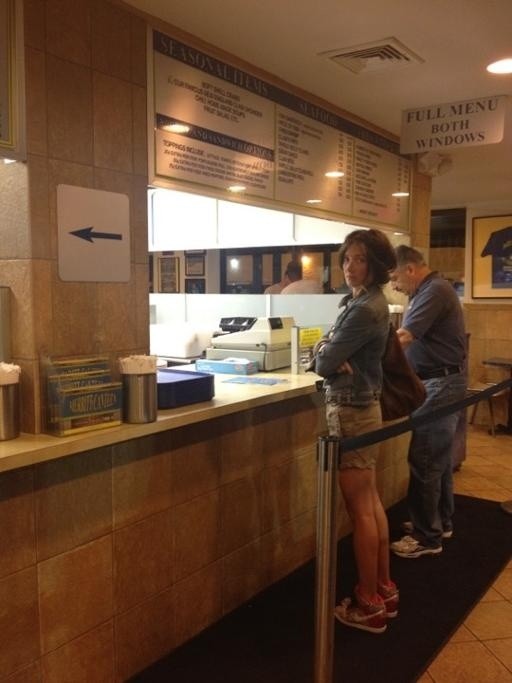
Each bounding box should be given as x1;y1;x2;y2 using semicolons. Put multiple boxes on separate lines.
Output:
148;249;207;293
471;213;512;300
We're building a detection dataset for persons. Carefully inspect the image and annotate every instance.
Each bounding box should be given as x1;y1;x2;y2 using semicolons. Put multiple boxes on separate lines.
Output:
305;229;400;633
280;261;325;294
388;245;468;559
264;271;290;294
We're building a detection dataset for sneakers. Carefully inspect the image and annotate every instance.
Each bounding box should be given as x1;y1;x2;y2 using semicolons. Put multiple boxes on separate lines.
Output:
334;579;400;634
389;518;456;560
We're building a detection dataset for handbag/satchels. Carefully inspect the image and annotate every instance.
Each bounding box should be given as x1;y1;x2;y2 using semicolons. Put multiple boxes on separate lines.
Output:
380;321;429;421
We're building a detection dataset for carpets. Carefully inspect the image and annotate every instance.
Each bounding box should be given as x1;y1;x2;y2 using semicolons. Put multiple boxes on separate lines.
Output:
118;491;512;682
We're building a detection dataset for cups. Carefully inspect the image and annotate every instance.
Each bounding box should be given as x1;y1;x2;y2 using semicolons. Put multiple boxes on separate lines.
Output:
388;312;402;330
122;373;158;424
0;382;21;441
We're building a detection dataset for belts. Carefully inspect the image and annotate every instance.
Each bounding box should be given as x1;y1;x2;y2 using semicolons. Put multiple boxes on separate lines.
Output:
413;362;467;380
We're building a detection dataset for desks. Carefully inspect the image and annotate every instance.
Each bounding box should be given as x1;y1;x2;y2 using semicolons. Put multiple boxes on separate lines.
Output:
484;358;512;438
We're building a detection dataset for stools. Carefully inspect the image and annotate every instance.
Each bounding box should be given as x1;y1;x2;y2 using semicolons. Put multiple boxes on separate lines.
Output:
466;379;505;437
